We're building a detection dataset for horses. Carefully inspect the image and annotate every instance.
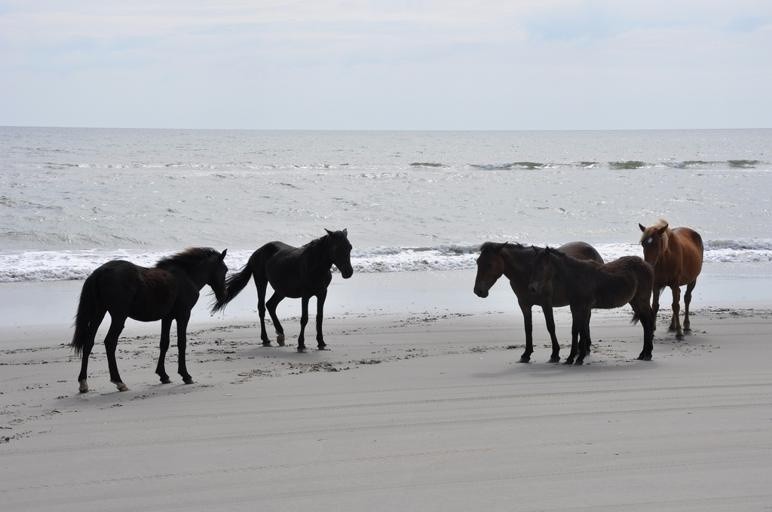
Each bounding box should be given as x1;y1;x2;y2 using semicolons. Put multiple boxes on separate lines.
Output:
636;221;704;344
474;242;613;366
72;247;230;395
529;243;656;365
213;228;353;354
